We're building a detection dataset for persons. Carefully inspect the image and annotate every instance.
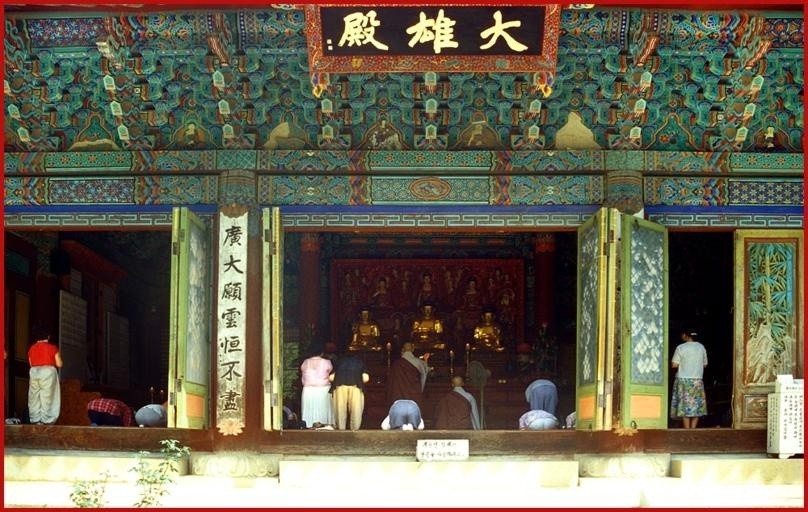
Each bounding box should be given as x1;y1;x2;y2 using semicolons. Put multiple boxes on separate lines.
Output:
471;306;506;352
518;377;576;430
433;375;482;430
669;324;709;429
27;317;63;425
86;397;168;428
300;342;371;431
410;300;447;350
369;264;517;316
347;306;383;352
381;341;429;430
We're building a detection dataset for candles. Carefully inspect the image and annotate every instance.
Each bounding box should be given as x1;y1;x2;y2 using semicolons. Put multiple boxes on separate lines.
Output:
465;342;470;372
386;342;391;367
449;351;454;374
